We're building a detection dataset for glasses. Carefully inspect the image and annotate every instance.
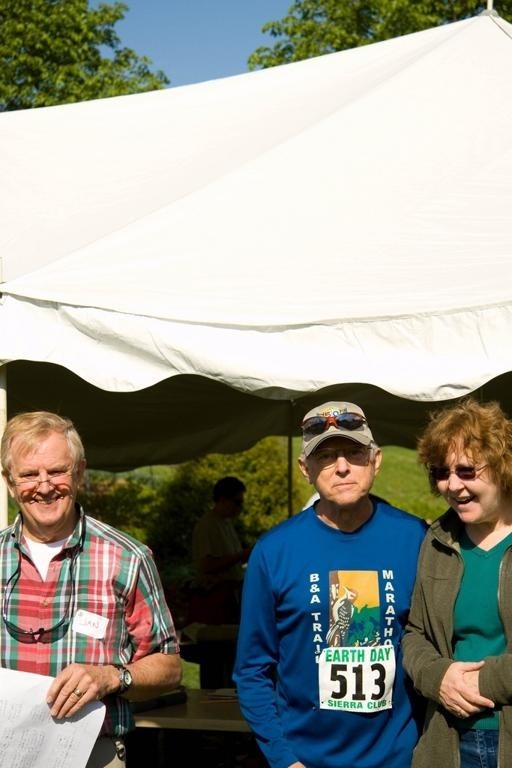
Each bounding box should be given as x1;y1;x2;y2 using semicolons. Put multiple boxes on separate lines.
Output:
420;459;492;480
301;411;371;434
15;475;69;491
2;585;76;642
314;443;371;466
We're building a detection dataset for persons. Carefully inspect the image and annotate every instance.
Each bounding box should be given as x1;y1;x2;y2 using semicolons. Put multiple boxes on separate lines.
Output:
232;401;432;768
402;398;512;768
195;476;249;624
1;412;183;768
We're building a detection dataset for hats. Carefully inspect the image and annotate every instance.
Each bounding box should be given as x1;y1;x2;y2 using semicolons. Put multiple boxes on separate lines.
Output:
301;401;376;458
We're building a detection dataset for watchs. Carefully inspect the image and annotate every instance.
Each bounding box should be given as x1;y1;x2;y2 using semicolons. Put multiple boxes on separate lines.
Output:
113;664;132;696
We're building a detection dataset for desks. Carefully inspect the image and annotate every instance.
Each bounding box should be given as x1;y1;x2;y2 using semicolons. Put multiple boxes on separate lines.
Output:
131;689;253;732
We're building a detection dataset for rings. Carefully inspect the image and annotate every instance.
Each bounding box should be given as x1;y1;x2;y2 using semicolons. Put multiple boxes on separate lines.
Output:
73;689;83;697
